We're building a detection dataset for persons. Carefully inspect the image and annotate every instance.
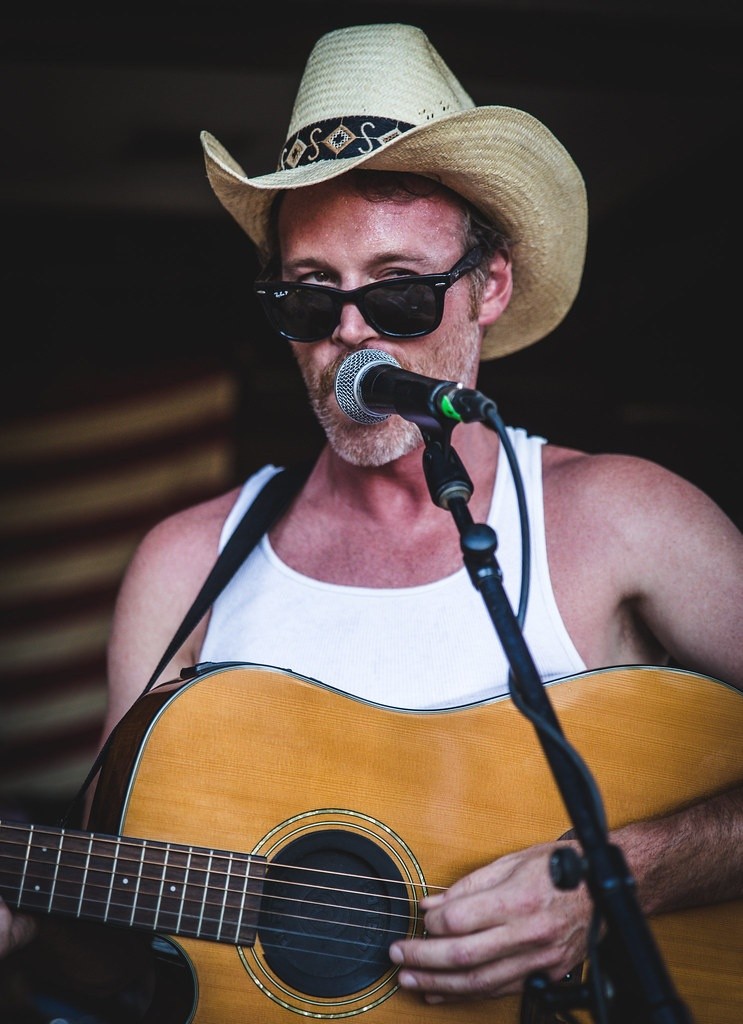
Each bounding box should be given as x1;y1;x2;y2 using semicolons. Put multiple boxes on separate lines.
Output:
0;24;743;1024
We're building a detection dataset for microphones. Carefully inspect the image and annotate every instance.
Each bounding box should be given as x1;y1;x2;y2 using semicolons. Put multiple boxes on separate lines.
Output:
334;350;496;425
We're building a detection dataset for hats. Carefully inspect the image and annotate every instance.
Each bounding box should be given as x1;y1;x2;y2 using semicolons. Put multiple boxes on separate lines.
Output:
198;22;590;359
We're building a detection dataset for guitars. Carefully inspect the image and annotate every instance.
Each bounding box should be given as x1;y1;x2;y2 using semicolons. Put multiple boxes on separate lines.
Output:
0;659;743;1023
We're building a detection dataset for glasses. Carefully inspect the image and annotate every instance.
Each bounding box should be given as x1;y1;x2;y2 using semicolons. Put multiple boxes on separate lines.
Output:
250;243;489;342
